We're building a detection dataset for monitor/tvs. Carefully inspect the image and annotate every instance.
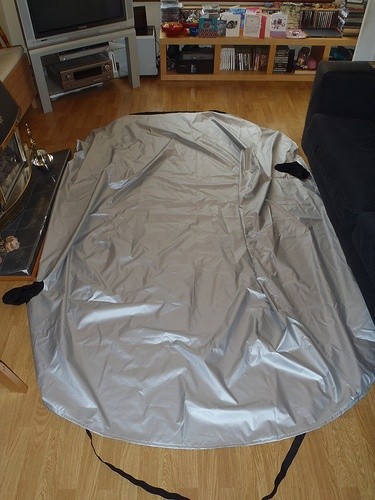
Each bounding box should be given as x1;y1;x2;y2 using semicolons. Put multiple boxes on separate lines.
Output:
15;0;135;51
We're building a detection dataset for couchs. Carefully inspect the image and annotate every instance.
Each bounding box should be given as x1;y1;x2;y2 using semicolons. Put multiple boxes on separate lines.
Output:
301;60;375;318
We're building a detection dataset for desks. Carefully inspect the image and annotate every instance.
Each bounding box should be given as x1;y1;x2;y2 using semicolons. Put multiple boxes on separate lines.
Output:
29;28;141;114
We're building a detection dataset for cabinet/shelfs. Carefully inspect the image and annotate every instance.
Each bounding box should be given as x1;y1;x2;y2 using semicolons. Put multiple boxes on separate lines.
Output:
0;45;38;151
159;27;357;81
